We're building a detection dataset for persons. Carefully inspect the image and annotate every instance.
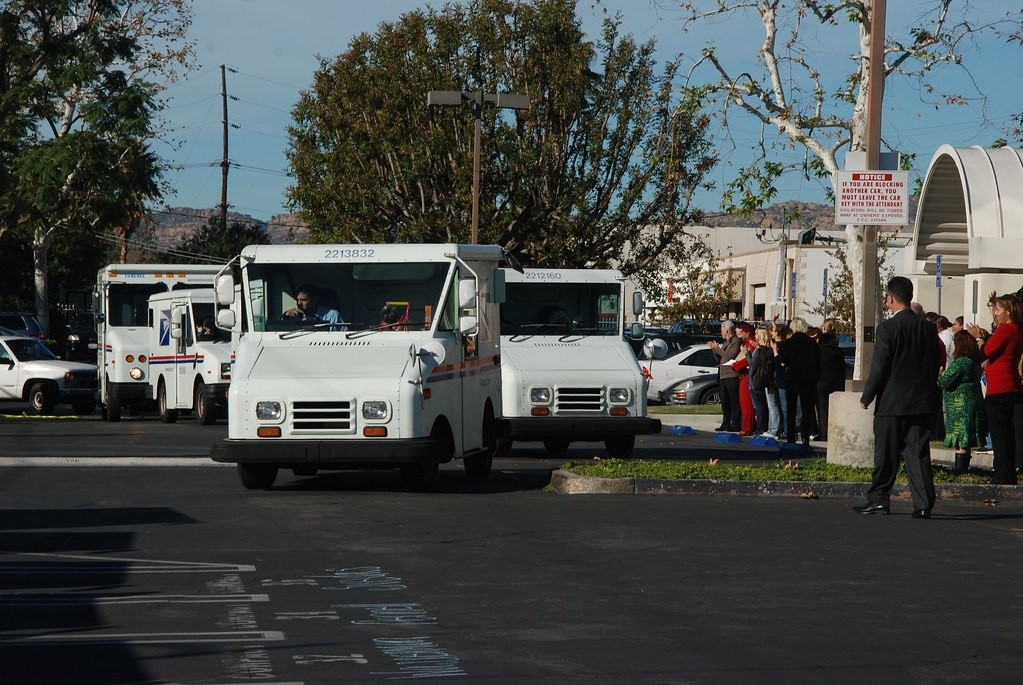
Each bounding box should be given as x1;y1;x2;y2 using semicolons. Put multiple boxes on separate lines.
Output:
280;284;348;331
197;317;214;335
706;313;846;445
911;285;1023;485
852;276;943;518
2;343;20;360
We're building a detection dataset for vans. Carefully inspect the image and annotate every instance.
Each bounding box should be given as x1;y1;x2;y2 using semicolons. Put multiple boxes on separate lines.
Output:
212;243;507;494
147;289;240;427
489;268;664;459
91;263;234;423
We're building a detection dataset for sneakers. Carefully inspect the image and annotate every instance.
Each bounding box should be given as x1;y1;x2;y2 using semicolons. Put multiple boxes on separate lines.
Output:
972;447;994;455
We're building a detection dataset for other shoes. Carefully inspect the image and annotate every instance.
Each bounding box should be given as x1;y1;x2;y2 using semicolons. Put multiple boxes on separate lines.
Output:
727;425;742;432
761;432;778;440
714;423;731;431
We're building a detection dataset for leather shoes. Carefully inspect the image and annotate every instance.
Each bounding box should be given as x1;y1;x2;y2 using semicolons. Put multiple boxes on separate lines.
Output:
912;509;931;518
852;502;890;515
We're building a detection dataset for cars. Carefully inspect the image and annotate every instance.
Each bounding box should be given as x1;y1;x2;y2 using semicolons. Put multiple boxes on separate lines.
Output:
628;318;769;402
657;374;722;409
838;334;856;370
65;311;97;359
0;311;45;343
0;335;98;415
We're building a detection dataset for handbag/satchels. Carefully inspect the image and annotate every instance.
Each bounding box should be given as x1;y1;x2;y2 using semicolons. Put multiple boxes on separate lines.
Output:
773;359;799;391
748;347;774;396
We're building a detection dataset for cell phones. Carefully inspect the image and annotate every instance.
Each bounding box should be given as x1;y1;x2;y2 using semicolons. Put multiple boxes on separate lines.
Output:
772;338;775;343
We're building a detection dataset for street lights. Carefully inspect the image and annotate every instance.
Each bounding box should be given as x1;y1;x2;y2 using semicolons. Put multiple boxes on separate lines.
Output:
425;88;532;245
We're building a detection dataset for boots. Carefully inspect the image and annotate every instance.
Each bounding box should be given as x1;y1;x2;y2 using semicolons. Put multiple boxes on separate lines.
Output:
954;453;970;473
